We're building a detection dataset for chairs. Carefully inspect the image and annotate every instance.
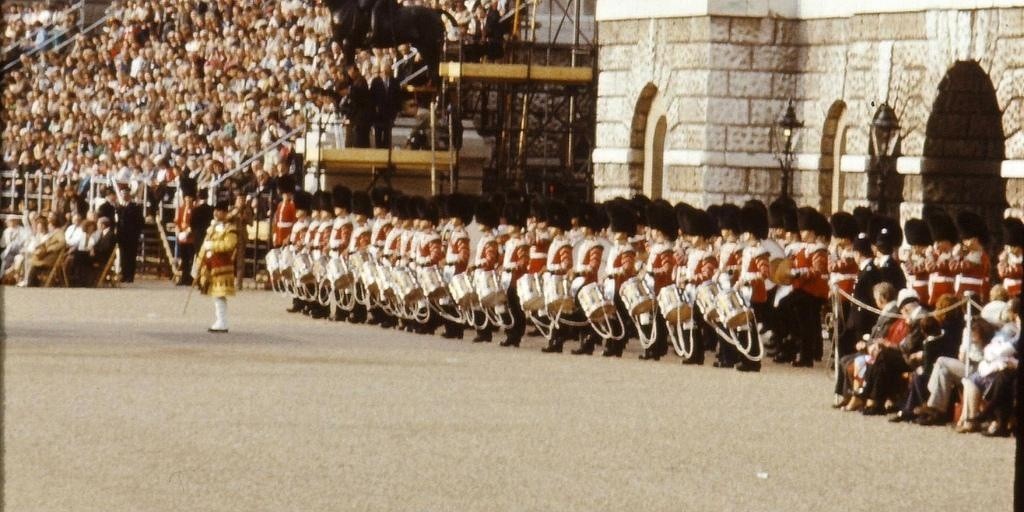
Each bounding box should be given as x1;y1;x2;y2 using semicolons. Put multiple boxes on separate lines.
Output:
32;242;119;288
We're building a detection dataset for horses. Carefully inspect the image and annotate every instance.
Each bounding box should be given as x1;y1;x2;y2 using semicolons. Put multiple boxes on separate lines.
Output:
307;0;459;88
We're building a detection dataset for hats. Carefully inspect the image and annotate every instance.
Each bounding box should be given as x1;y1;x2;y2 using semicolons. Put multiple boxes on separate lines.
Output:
431;191;832;238
295;184;436;222
830;206;1024;251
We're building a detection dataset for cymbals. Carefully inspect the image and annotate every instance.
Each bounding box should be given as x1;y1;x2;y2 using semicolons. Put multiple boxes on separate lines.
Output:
776;259;795;285
768;258;783;283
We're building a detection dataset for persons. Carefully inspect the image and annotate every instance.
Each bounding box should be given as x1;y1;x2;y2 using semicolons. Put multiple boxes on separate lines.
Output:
173;179;253;294
0;185;146;285
193;202;238;332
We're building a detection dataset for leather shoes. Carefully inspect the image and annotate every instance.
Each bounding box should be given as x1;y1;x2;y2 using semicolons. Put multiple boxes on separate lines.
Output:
540;337;670;360
832;395;1015;437
441;326;523;348
681;337;816;373
286;299;438;337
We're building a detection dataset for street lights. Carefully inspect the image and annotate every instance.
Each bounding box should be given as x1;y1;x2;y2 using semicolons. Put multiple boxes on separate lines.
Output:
869;103;902;220
779;104;805;243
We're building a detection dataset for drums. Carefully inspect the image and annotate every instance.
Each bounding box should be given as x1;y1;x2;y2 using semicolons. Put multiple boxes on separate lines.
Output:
618;276;658;349
348;251;382;310
543;274;575;329
448;269;490;329
375;266;414;320
265;248;289;297
693;279;741;345
389;266;430;324
279;248;309;298
417;264;467;325
657;283;695;359
577;281;625;340
292;253;330;303
516;271;555;341
475;269;515;328
311;256;345;307
713;289;764;362
324;257;356;312
